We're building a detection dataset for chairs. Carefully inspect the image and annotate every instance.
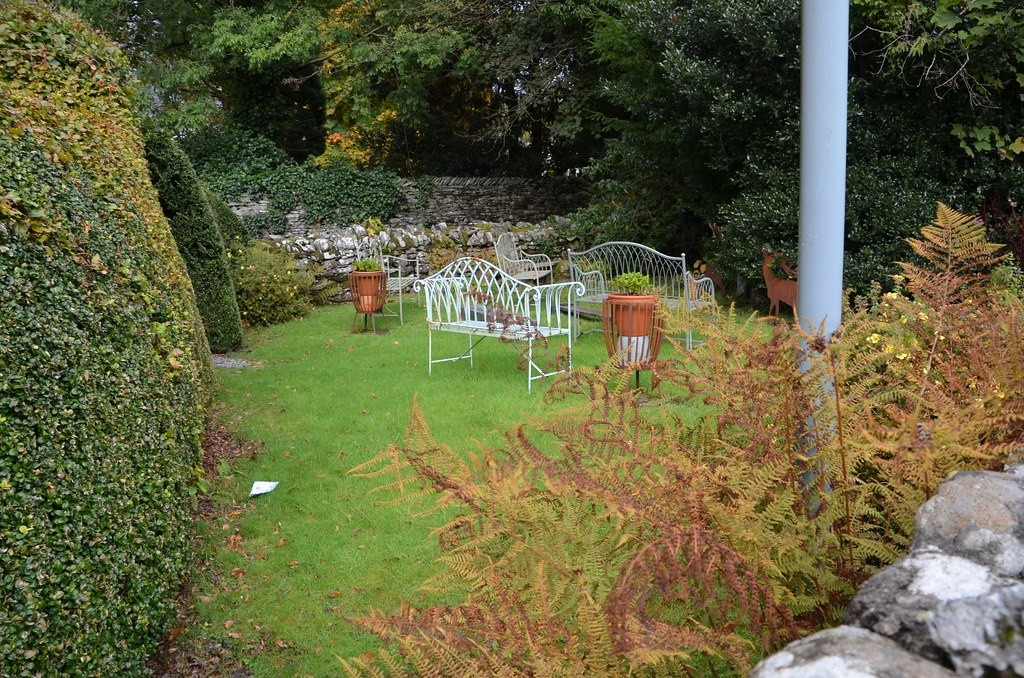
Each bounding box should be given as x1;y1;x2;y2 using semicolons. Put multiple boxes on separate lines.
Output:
358;236;420;327
494;232;554;307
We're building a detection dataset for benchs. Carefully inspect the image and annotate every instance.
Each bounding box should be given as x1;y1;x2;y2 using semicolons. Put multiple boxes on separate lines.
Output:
413;256;586;394
567;240;716;354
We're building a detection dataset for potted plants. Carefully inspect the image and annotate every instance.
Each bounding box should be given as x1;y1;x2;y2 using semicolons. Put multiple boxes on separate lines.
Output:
601;271;667;394
348;258;392;336
